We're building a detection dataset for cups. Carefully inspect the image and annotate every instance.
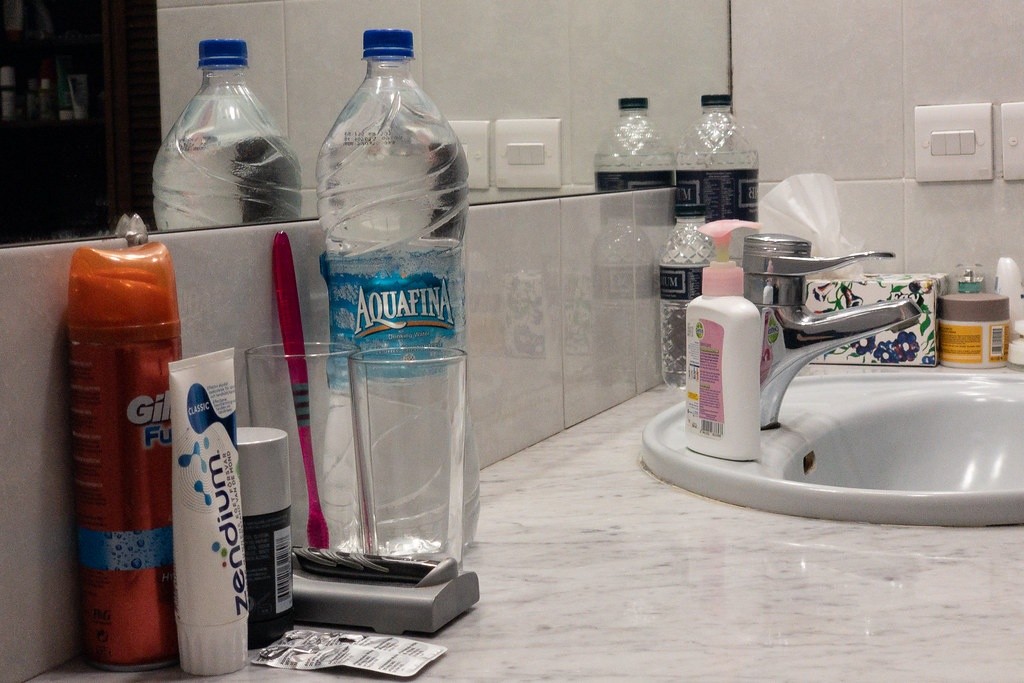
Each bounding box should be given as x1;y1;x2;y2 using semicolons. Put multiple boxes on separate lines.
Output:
244;342;377;570
348;346;469;576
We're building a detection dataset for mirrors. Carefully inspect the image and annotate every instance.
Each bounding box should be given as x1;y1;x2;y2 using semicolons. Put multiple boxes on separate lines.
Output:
0;0;734;252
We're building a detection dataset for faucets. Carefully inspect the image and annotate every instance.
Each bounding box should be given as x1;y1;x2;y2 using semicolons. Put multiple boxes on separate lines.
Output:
743;234;921;429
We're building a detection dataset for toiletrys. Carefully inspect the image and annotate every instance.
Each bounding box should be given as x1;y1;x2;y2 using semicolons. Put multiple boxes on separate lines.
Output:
937;258;1021;370
685;219;761;464
66;245;293;677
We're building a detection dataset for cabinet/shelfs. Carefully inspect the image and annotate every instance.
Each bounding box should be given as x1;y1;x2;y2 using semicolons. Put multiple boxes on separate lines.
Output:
0;0;117;244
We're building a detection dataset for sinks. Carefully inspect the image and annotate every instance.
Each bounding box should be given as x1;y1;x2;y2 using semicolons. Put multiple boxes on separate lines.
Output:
771;391;1024;493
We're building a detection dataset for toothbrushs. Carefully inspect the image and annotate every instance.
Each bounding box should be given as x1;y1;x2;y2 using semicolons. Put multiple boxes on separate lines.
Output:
272;230;330;550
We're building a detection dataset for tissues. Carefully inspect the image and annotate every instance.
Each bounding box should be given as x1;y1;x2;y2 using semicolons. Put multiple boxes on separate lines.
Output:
761;175;951;367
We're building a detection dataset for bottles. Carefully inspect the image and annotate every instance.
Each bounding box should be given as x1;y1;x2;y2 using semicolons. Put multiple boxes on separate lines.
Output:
657;202;715;389
151;38;305;234
939;293;1011;369
595;97;676;193
0;67;17;121
315;30;480;566
673;95;760;267
26;77;55;118
235;426;294;651
65;242;184;673
